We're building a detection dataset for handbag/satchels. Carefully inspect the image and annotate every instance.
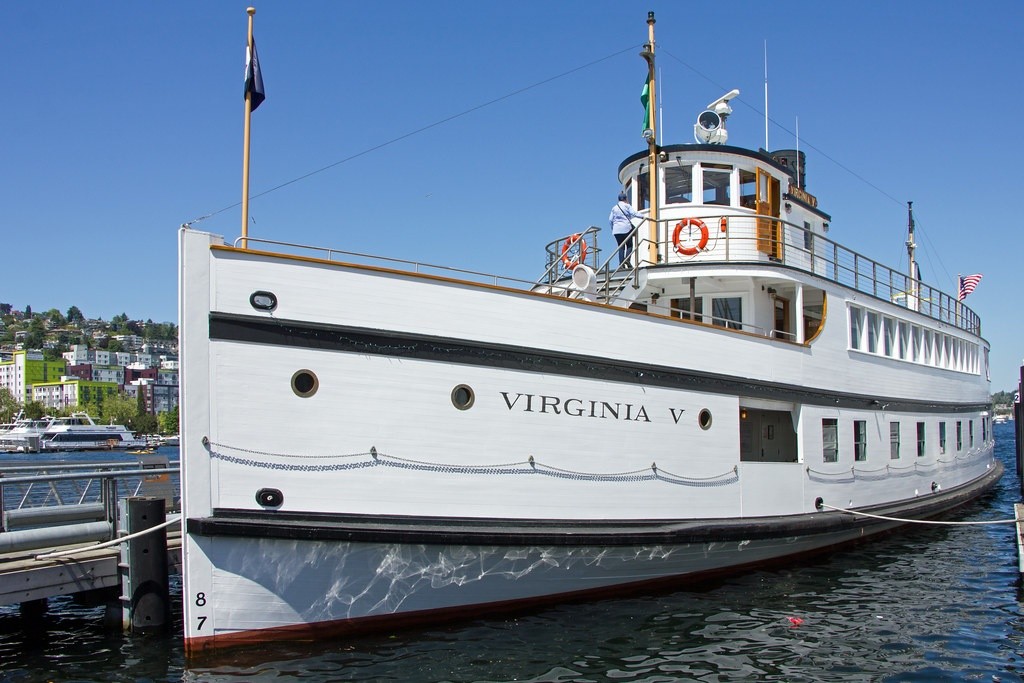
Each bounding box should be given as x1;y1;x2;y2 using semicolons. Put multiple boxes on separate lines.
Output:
628;223;639;237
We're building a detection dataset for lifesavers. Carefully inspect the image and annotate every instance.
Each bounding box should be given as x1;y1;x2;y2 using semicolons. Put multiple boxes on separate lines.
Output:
560;234;588;267
672;217;709;255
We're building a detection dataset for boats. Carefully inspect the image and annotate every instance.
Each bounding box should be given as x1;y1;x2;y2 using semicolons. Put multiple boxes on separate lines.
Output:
176;5;1005;661
0;409;138;454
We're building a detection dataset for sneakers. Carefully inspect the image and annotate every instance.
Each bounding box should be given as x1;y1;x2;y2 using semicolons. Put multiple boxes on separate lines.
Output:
624;260;634;268
619;264;625;270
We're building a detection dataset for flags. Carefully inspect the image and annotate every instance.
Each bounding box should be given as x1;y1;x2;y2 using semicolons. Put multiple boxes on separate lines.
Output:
960;274;983;301
244;36;268;112
639;73;650;134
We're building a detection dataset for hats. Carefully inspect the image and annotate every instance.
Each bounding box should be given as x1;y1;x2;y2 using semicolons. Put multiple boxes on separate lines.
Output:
619;193;627;199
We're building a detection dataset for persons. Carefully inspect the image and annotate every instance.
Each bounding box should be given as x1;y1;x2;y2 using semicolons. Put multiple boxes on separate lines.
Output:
609;193;644;269
